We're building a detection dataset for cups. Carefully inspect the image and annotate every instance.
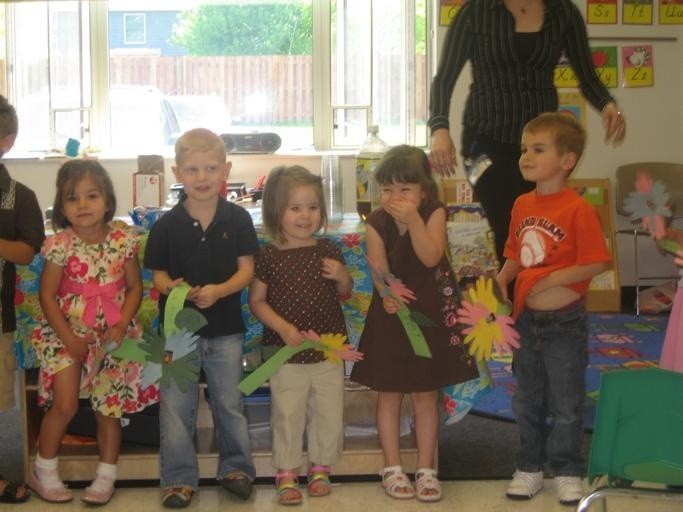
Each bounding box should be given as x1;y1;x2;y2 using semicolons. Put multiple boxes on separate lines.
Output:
319;152;343;226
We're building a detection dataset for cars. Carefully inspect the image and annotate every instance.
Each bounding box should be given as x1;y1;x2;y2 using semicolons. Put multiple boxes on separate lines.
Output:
109;86;192;157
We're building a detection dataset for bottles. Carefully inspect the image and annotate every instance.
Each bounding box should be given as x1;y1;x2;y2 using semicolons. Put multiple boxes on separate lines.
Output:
354;122;387;224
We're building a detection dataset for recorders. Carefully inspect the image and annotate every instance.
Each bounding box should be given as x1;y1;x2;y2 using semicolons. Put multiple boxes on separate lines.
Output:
218;133;281;154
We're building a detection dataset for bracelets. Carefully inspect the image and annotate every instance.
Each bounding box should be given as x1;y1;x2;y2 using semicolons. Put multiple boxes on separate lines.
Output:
336;295;352;301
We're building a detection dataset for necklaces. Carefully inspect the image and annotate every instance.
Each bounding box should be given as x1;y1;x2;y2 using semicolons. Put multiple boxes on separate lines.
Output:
514;2;530;14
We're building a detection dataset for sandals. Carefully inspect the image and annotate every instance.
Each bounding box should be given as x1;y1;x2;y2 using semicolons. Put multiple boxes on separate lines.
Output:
275;472;303;504
378;465;415;499
2;474;31;502
415;467;442;501
306;466;332;495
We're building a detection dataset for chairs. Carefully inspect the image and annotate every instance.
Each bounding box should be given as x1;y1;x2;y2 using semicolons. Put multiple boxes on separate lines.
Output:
574;365;683;512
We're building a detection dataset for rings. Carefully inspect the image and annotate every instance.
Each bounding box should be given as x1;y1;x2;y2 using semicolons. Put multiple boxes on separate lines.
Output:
617;111;622;116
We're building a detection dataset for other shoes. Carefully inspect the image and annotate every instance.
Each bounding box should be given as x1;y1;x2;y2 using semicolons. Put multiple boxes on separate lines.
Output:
27;470;74;502
81;473;117;503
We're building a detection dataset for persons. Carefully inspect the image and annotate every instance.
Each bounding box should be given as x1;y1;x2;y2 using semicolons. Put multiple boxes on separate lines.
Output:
248;165;355;504
143;127;257;509
424;1;627;303
0;95;46;502
493;110;612;507
26;158;144;507
347;143;481;504
658;244;682;377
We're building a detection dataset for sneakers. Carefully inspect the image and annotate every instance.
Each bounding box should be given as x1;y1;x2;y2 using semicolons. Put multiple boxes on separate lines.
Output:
553;475;582;502
160;486;193;507
506;467;544;497
220;473;253;494
